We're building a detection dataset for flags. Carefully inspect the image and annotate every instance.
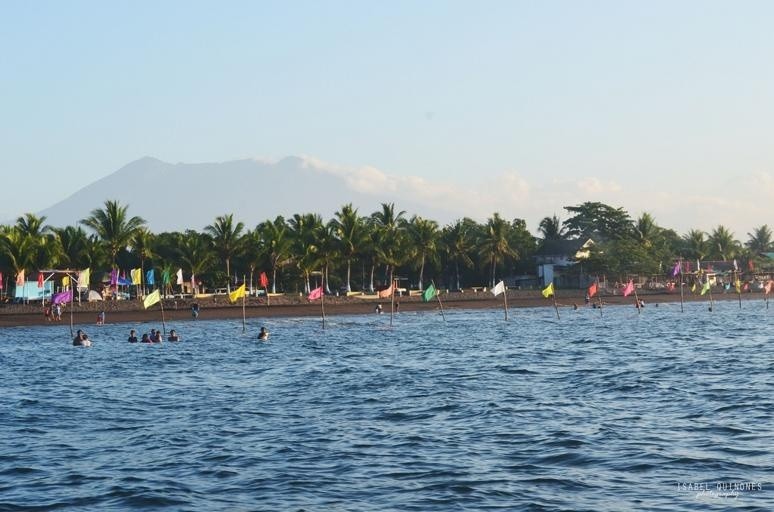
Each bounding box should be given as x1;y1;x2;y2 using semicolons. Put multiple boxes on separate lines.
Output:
585;259;774;298
3;266;560;308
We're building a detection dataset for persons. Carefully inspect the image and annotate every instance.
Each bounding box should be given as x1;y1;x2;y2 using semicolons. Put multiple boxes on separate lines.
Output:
72;329;91;347
192;302;199;322
571;291;646;311
376;300;400;314
42;303;61;322
127;328;180;344
96;310;105;325
258;326;269;341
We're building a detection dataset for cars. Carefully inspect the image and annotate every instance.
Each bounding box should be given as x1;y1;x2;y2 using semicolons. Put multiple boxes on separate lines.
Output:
111;285;265;302
